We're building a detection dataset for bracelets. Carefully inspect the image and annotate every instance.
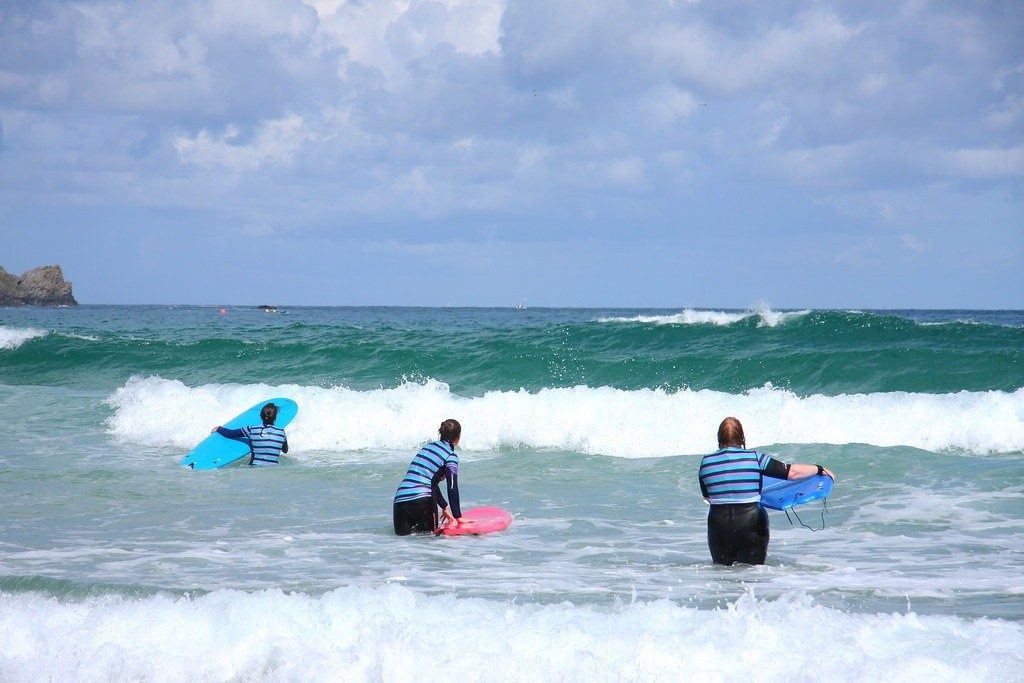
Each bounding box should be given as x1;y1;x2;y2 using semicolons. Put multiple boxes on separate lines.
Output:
816;465;823;476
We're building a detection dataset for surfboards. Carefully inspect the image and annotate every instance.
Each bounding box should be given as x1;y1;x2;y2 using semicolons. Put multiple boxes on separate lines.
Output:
183;397;298;469
760;475;832;510
439;504;512;536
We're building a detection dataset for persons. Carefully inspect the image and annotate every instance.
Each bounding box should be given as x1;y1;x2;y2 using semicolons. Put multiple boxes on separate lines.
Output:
211;403;288;467
393;419;479;536
699;417;835;566
